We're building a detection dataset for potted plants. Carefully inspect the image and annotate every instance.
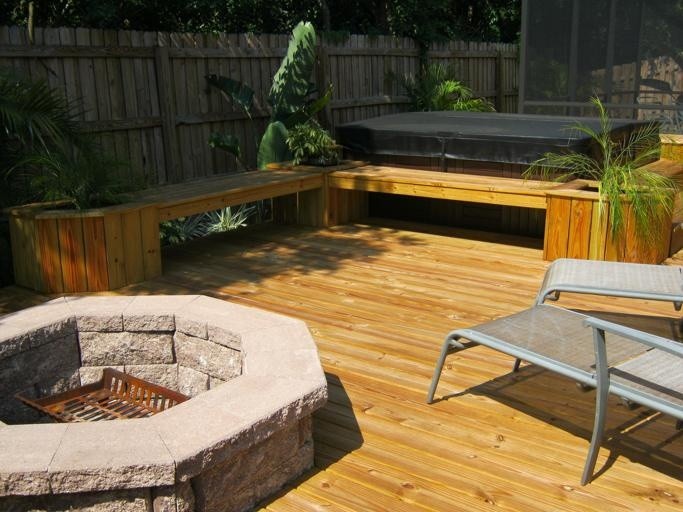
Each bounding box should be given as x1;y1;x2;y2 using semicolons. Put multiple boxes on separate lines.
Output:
3;126;161;297
267;125;370;228
520;95;677;266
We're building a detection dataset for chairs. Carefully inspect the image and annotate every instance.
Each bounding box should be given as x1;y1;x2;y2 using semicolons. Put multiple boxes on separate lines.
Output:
534;260;682;317
428;302;683;485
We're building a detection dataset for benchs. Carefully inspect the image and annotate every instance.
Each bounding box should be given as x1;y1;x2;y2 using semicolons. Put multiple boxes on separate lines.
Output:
323;165;566;229
115;170;328;227
